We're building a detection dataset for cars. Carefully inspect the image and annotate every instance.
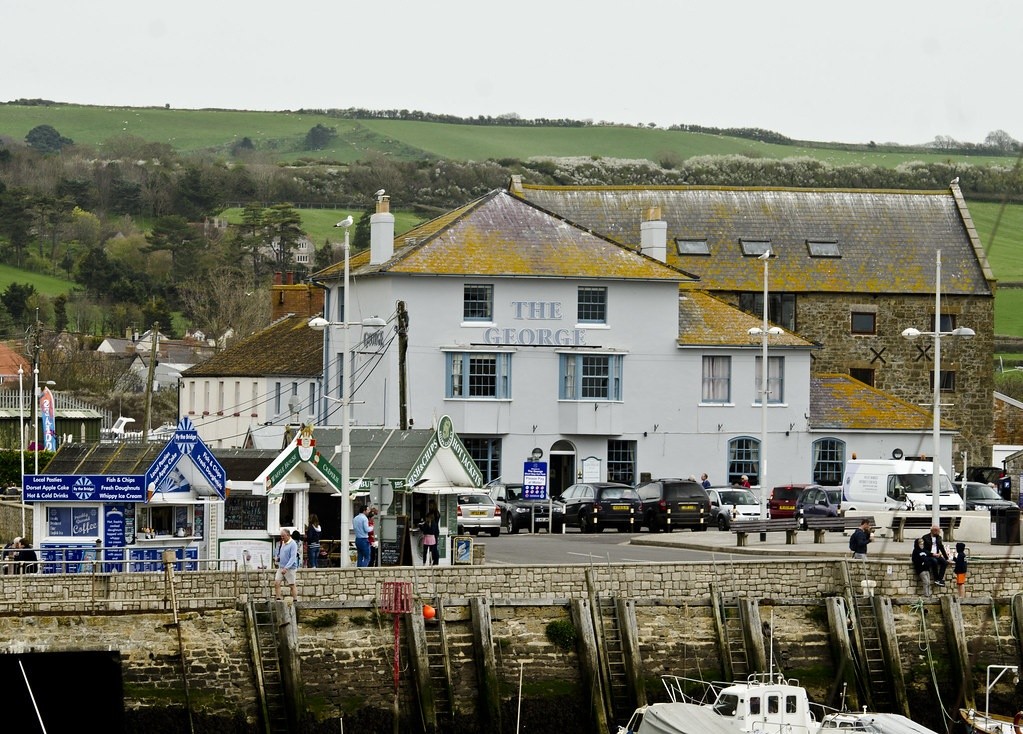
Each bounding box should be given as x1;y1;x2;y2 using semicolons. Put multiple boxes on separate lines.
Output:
793;484;843;532
487;482;565;535
766;485;822;520
703;487;772;532
456;493;504;536
951;466;1020;517
552;482;645;535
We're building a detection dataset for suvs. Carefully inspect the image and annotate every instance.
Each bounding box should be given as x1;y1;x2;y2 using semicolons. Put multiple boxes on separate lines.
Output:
634;478;714;532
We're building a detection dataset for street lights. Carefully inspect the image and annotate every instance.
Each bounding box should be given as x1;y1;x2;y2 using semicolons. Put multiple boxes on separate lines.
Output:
900;326;976;543
29;377;57;447
308;316;390;568
746;325;785;530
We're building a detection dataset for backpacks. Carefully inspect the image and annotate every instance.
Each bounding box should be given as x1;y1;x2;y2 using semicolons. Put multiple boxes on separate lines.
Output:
850;530;866;551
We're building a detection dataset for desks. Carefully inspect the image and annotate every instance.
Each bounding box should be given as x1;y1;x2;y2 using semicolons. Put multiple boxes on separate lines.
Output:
319;539;341;567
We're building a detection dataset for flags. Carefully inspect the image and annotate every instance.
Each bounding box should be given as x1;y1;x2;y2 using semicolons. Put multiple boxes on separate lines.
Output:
39;386;56;452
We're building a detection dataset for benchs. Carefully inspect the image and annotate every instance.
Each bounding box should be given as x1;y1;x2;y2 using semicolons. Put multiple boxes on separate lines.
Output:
729;517;799;547
886;517;963;543
805;516;882;545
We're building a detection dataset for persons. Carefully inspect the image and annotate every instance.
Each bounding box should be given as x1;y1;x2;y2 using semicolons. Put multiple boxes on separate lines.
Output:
305;531;320;568
275;528;297;602
701;473;712;495
854;520;875;558
2;537;38;574
291;531;302;568
988;482;994;488
922;525;952;587
418;512;439;565
949;543;967;598
912;538;932;597
739;475;750;497
429;499;440;546
307;513;324;541
367;514;382;566
352;505;374;567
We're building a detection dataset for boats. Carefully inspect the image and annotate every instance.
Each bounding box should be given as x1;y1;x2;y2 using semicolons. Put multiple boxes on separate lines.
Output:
958;665;1023;734
615;608;938;734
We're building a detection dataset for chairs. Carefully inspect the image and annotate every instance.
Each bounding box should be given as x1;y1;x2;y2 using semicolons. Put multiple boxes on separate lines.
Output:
25;560;47;574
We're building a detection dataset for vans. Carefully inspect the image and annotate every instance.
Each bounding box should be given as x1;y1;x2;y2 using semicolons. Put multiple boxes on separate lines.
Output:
840;460;964;512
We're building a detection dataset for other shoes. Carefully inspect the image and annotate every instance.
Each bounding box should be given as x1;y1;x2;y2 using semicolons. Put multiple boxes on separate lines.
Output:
934;579;945;586
